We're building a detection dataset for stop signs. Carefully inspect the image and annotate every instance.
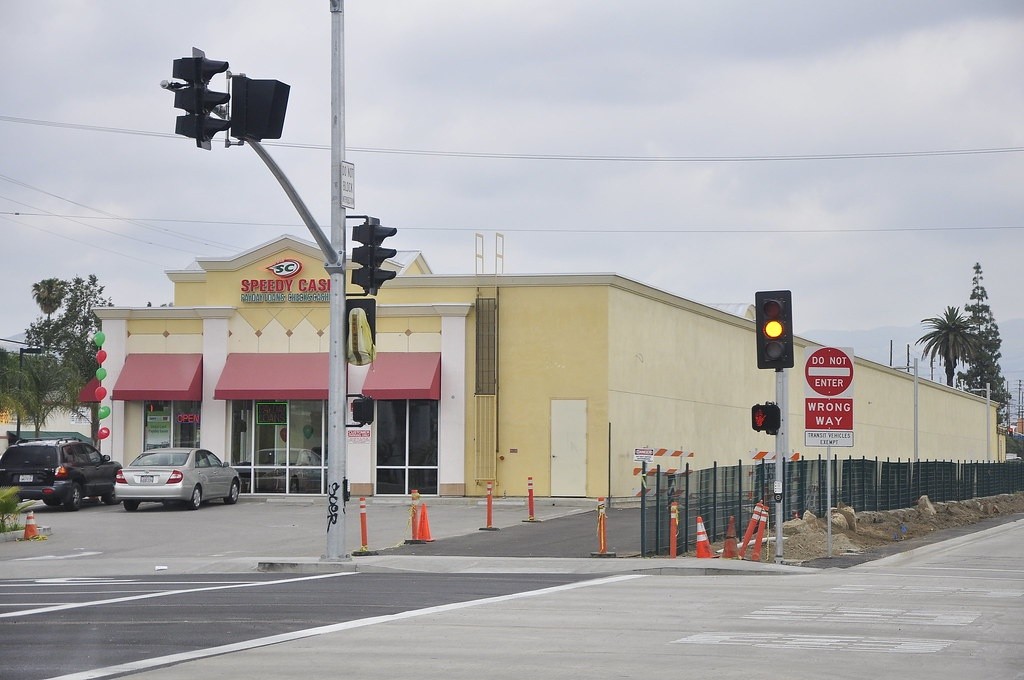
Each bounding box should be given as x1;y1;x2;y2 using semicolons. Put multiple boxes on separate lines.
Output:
803;346;855;399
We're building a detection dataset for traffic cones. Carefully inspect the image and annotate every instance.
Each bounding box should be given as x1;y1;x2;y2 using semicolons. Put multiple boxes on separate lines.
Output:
719;516;738;559
418;502;436;542
23;509;39;540
696;516;714;558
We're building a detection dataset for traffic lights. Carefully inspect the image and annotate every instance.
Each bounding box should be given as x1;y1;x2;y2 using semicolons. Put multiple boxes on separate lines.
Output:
172;57;233;151
352;223;398;294
755;290;794;369
751;401;781;437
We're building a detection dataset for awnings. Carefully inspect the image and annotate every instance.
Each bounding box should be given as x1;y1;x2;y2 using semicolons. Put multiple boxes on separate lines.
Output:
362;352;441;401
111;353;203;402
213;352;348;400
78;376;102;404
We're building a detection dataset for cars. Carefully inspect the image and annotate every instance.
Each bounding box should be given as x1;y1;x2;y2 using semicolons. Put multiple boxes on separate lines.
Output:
239;448;328;492
115;446;241;511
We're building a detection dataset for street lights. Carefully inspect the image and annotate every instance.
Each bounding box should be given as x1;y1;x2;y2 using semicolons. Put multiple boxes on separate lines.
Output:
16;347;43;441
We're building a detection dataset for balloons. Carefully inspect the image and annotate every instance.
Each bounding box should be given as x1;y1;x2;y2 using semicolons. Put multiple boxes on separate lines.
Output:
96;350;106;364
97;406;110;420
302;424;313;439
93;331;106;347
95;387;107;401
280;428;287;443
97;427;110;440
95;368;107;381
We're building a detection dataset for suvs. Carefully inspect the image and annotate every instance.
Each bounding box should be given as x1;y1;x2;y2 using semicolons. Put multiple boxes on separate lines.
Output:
0;437;122;511
